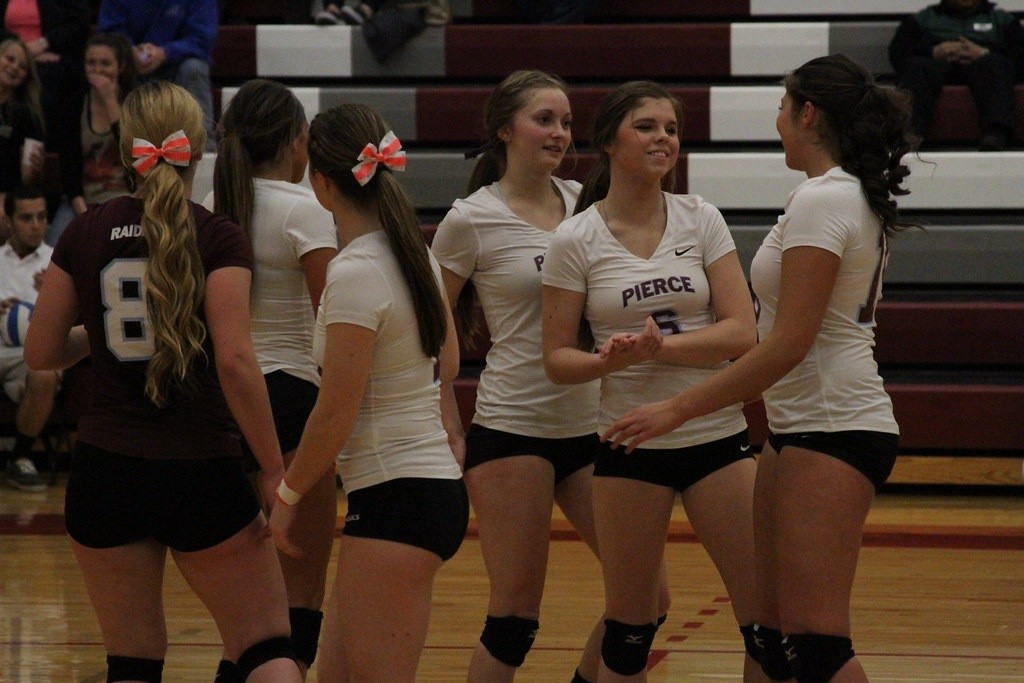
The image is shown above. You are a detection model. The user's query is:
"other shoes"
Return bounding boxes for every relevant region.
[340,6,367,26]
[313,10,336,23]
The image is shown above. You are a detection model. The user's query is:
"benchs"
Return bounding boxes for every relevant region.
[190,0,1024,460]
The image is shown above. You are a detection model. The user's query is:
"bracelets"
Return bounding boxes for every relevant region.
[274,479,301,506]
[982,47,990,56]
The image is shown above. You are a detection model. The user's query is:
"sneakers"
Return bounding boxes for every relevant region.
[7,458,48,490]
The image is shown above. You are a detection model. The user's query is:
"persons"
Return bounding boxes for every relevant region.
[0,0,1024,683]
[600,55,916,683]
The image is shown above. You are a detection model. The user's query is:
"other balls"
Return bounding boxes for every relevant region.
[0,300,35,347]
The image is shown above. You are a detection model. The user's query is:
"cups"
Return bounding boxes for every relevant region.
[22,138,43,165]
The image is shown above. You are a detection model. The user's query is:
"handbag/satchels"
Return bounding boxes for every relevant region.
[365,0,448,62]
